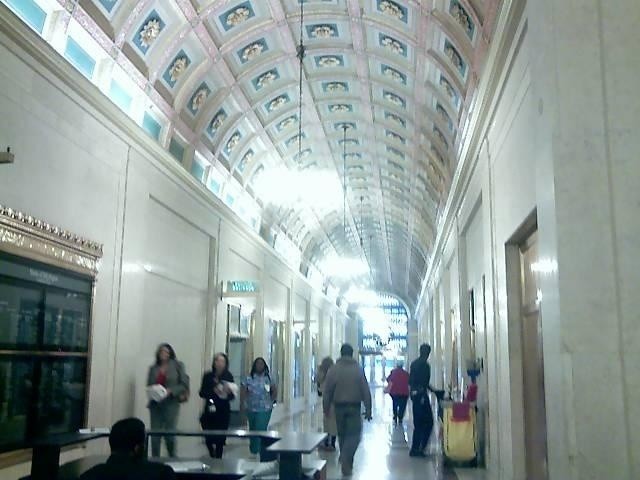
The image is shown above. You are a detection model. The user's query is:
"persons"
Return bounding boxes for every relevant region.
[386,360,410,424]
[408,343,437,458]
[322,343,372,476]
[316,357,338,449]
[198,352,234,459]
[145,343,189,459]
[82,418,176,479]
[241,357,278,458]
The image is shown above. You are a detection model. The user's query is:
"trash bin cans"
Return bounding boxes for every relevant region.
[438,398,478,468]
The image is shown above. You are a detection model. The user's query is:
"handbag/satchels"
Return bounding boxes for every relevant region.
[175,361,189,403]
